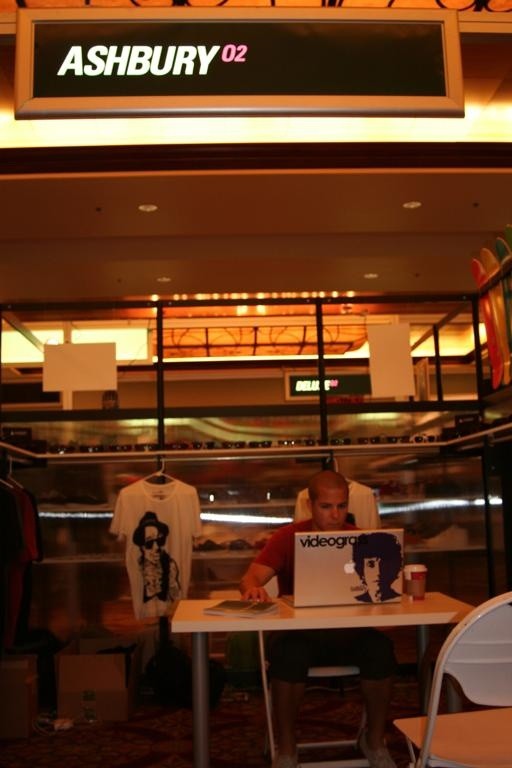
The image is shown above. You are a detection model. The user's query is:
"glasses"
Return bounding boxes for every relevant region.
[356,436,382,444]
[329,438,353,445]
[136,443,160,452]
[140,534,166,549]
[79,444,105,453]
[414,435,436,443]
[384,435,410,443]
[165,443,189,450]
[50,444,75,454]
[191,441,215,449]
[277,440,300,446]
[304,439,327,446]
[110,445,132,452]
[221,441,246,449]
[248,441,273,448]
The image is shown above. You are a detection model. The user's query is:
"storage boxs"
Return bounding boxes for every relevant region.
[1,634,133,744]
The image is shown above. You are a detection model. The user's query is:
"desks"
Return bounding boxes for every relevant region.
[170,588,475,767]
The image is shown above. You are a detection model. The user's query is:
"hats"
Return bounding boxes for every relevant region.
[131,510,170,547]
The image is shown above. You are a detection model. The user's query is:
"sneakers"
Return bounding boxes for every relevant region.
[357,727,398,767]
[191,538,254,551]
[269,746,301,767]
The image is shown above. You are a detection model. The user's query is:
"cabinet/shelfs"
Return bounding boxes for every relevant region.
[2,412,512,572]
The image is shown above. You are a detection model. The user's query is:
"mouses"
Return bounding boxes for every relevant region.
[248,598,267,604]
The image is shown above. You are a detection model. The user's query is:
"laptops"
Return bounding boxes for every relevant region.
[282,527,405,610]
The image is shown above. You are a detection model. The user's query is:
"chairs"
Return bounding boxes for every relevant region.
[390,587,512,767]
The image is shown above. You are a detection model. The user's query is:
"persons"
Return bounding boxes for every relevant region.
[240,469,402,768]
[131,510,181,601]
[352,531,402,602]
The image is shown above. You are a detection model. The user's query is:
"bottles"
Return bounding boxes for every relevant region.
[79,672,97,723]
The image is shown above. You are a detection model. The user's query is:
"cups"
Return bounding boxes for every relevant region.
[403,564,428,603]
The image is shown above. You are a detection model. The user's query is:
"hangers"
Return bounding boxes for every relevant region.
[0,452,23,486]
[140,457,176,489]
